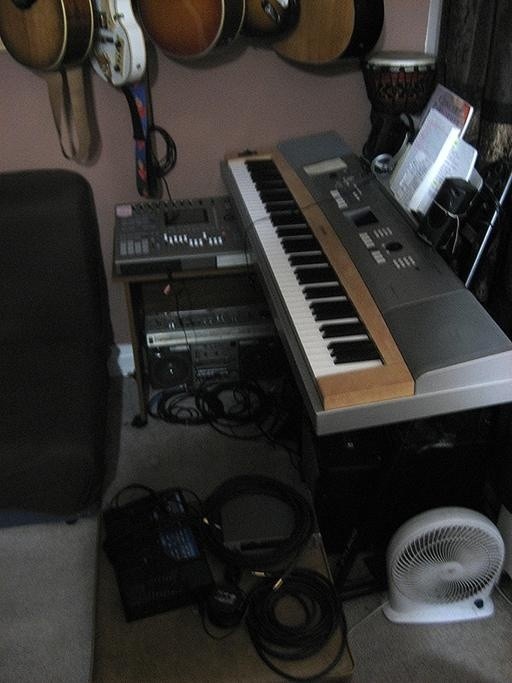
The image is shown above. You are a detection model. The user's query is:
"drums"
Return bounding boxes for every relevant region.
[361,51,436,164]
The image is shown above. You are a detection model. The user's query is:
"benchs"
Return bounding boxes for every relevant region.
[0,169,123,528]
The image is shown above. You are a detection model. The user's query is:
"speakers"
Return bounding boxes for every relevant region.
[417,178,477,248]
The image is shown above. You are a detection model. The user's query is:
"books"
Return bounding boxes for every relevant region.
[448,138,479,181]
[388,107,460,209]
[388,83,473,214]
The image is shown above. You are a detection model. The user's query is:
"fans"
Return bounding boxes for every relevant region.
[379,506,506,625]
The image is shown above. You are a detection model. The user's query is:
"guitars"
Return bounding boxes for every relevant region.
[0,0,383,88]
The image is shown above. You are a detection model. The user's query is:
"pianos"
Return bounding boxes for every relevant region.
[220,129,512,437]
